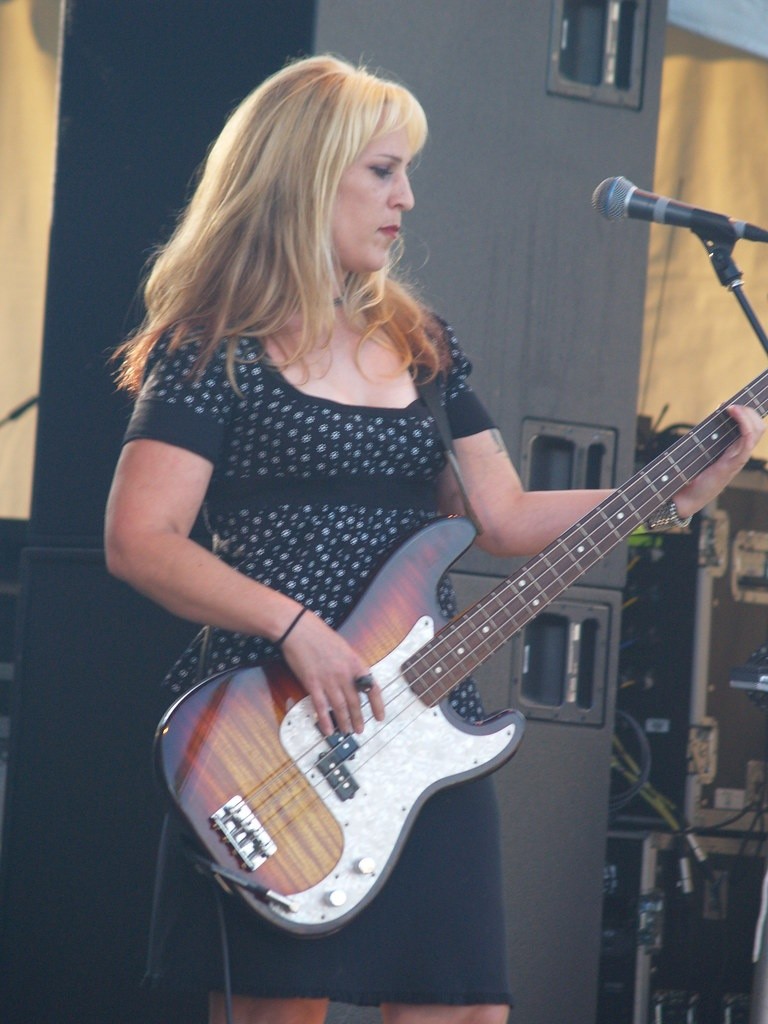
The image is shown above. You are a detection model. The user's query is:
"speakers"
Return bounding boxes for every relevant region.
[28,0,665,591]
[0,546,624,1024]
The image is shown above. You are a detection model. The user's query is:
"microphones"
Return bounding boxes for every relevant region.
[592,176,768,243]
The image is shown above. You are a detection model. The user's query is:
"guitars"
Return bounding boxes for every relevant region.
[146,369,766,939]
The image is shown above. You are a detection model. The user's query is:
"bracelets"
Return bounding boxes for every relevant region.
[642,497,694,529]
[273,608,309,647]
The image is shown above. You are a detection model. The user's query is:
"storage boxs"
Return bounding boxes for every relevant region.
[611,465,768,837]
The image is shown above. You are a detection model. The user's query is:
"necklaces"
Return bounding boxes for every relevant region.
[334,297,344,307]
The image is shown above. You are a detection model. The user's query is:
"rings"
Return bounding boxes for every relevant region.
[357,674,375,690]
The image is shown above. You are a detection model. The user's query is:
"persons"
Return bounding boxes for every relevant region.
[102,57,768,1024]
